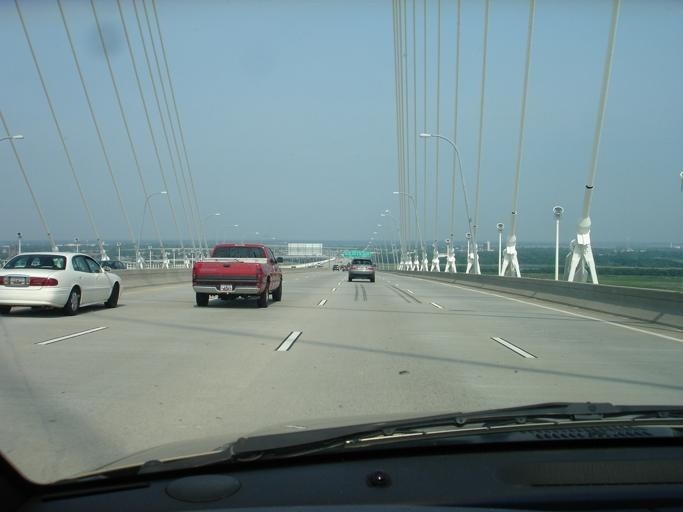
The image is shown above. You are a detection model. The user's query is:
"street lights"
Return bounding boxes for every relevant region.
[136,191,168,269]
[419,134,478,274]
[198,213,220,257]
[365,192,423,270]
[0,135,24,142]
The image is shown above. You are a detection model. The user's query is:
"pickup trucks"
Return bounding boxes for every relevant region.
[192,244,283,308]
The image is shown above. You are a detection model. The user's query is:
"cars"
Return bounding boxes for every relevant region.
[333,259,376,282]
[0,252,122,316]
